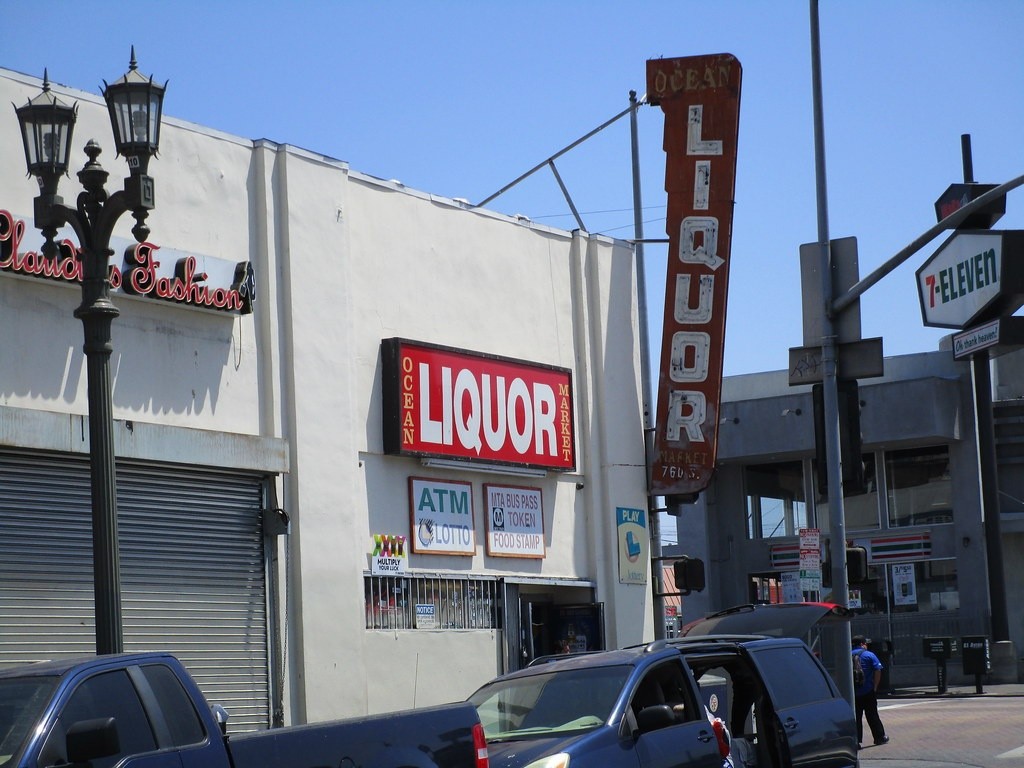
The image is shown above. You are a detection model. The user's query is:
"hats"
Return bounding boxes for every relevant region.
[851,635,872,645]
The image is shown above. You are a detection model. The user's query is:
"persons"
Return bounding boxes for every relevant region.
[850,633,890,748]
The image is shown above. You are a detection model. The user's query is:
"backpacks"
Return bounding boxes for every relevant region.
[853,649,866,689]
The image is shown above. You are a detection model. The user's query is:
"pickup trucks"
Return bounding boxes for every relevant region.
[0,649,488,768]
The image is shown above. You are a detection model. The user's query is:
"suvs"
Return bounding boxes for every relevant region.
[467,601,860,768]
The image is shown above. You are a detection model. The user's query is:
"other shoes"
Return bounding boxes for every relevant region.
[873,735,890,745]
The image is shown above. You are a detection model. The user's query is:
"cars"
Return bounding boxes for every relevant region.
[665,616,681,631]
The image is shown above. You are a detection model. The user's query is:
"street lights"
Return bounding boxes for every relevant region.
[9,40,170,655]
